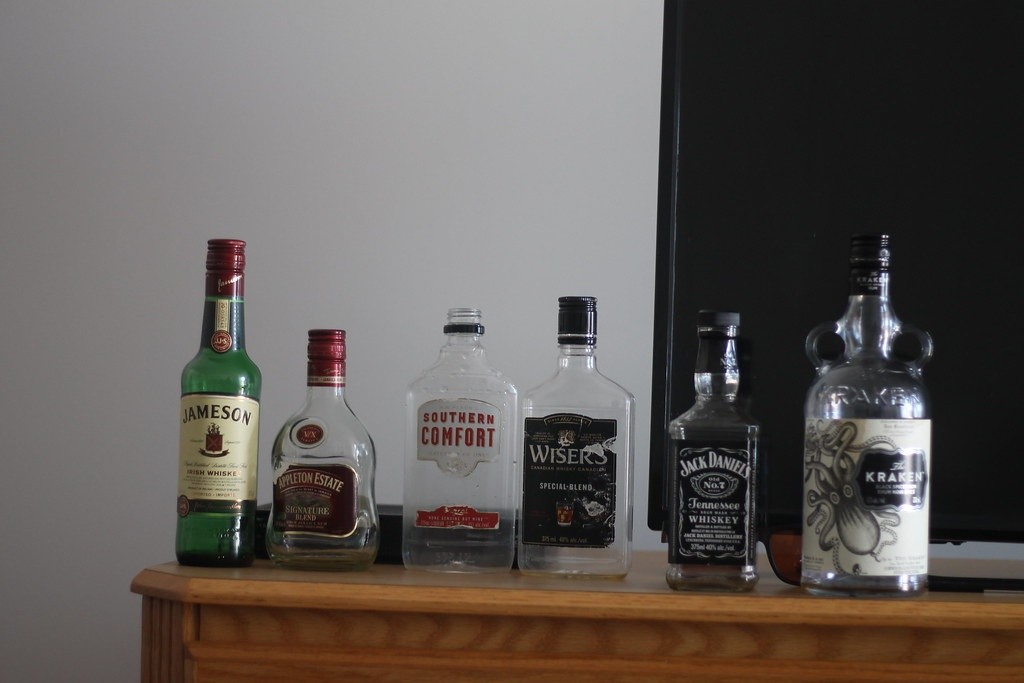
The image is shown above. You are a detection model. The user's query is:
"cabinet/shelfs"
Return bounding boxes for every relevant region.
[130,548,1024,683]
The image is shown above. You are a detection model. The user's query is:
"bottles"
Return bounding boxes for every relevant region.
[176,234,934,599]
